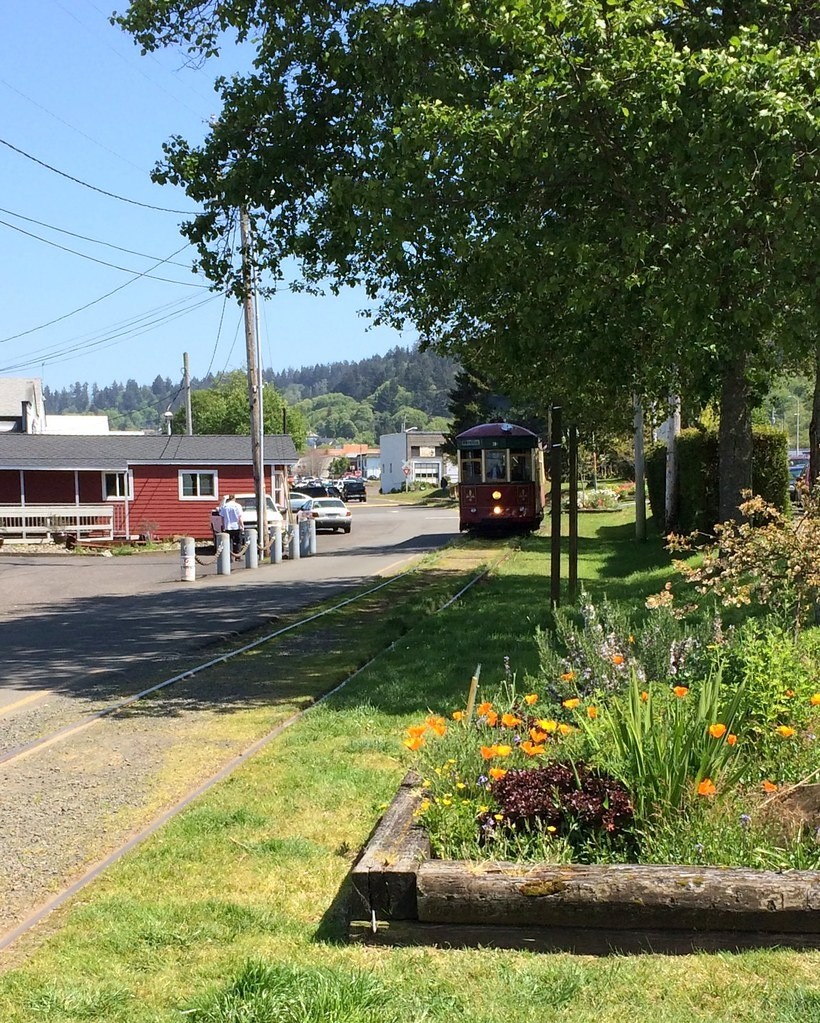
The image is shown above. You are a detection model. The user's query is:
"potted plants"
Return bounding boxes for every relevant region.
[45,514,69,544]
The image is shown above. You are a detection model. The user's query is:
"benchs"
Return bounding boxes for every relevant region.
[0,506,114,545]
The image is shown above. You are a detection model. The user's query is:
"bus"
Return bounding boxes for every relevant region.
[453,423,545,537]
[789,455,810,490]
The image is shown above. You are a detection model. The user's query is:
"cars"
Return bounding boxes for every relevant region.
[296,500,352,534]
[788,465,804,498]
[288,492,321,512]
[288,476,357,499]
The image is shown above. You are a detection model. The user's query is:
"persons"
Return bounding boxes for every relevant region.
[219,494,244,561]
[441,477,448,497]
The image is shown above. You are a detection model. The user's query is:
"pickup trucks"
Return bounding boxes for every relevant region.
[210,493,286,554]
[341,484,366,502]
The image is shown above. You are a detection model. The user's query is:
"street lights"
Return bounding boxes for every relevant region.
[163,412,174,434]
[787,395,799,456]
